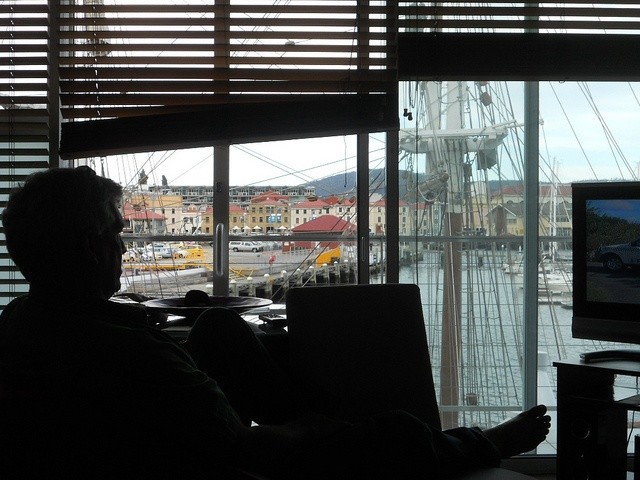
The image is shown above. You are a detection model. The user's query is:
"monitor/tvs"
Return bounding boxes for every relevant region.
[568,180,640,364]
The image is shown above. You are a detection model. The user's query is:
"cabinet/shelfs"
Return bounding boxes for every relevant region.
[552,351,636,480]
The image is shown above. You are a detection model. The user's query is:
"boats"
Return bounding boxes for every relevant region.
[503,249,575,310]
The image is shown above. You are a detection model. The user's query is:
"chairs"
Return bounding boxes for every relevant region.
[286,283,535,480]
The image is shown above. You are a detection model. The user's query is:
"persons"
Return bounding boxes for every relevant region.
[0,165,553,479]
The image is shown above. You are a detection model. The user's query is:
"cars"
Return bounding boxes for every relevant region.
[595,239,640,273]
[122,241,202,262]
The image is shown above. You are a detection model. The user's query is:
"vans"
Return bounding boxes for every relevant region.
[230,241,262,253]
